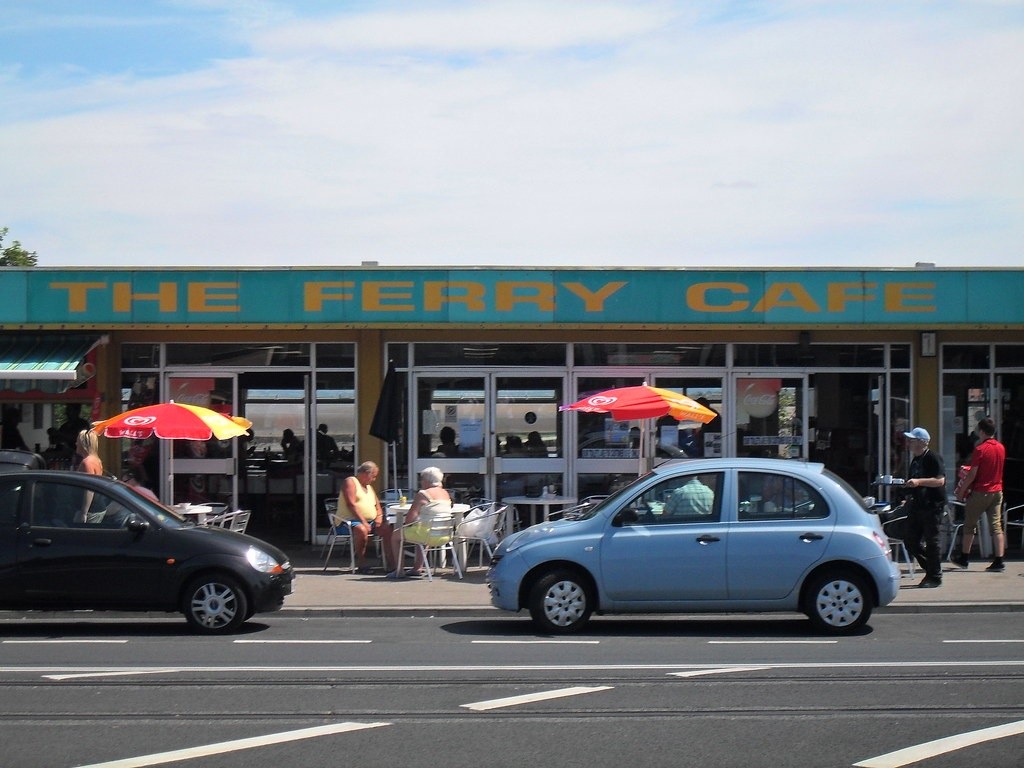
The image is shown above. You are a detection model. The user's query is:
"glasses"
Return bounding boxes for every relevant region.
[904,437,918,443]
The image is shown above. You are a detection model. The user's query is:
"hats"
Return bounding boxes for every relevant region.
[904,427,930,441]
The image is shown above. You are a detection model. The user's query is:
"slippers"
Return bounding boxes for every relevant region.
[386,570,405,578]
[406,568,423,576]
[358,566,372,575]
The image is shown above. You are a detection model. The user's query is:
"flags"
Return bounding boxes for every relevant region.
[370,360,404,447]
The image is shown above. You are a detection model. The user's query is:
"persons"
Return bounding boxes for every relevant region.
[316,423,349,464]
[44,402,90,459]
[386,466,453,582]
[902,425,949,588]
[280,429,304,460]
[694,397,722,457]
[660,472,715,516]
[523,429,549,459]
[432,426,474,484]
[332,458,394,572]
[0,407,31,451]
[237,428,257,464]
[950,416,1006,573]
[497,434,543,497]
[71,428,109,524]
[466,430,503,496]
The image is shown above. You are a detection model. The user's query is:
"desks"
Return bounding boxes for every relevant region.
[175,505,213,524]
[502,495,578,526]
[227,475,335,494]
[388,503,470,575]
[636,501,665,515]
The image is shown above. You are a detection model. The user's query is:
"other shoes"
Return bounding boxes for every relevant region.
[952,558,968,569]
[986,563,1006,572]
[918,575,942,588]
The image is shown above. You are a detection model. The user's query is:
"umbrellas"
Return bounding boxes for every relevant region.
[558,381,717,477]
[91,396,254,506]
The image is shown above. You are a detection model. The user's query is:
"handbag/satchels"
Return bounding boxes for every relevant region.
[75,470,117,513]
[459,503,501,548]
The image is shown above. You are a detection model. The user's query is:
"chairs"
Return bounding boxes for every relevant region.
[197,460,354,534]
[947,501,1024,563]
[546,495,611,521]
[882,509,916,580]
[319,488,523,582]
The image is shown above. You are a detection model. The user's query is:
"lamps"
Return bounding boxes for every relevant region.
[798,332,815,359]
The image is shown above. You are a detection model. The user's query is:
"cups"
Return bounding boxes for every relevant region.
[399,496,406,507]
[178,503,192,510]
[874,474,905,484]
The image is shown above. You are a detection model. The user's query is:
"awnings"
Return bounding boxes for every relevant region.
[0,332,111,381]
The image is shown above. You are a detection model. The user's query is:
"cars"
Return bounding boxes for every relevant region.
[485,457,901,635]
[501,430,687,526]
[0,471,296,635]
